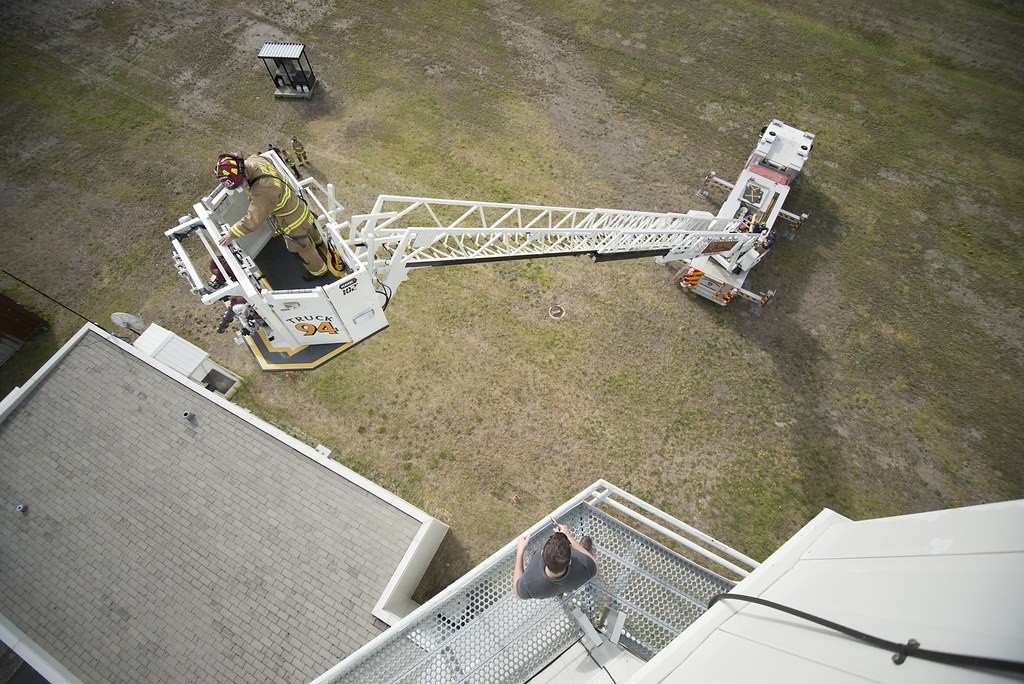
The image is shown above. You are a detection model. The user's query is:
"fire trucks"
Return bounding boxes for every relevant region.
[164,117,817,349]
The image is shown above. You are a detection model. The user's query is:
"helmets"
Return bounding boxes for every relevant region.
[215,152,244,190]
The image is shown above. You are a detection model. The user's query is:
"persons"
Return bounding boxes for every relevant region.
[513,523,600,600]
[282,150,301,179]
[291,136,309,167]
[268,144,281,154]
[214,152,327,281]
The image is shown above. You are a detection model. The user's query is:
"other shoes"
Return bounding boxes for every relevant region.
[302,270,326,280]
[299,163,303,167]
[297,175,300,178]
[579,536,592,551]
[306,161,309,164]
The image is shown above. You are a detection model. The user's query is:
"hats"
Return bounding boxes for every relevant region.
[291,136,298,142]
